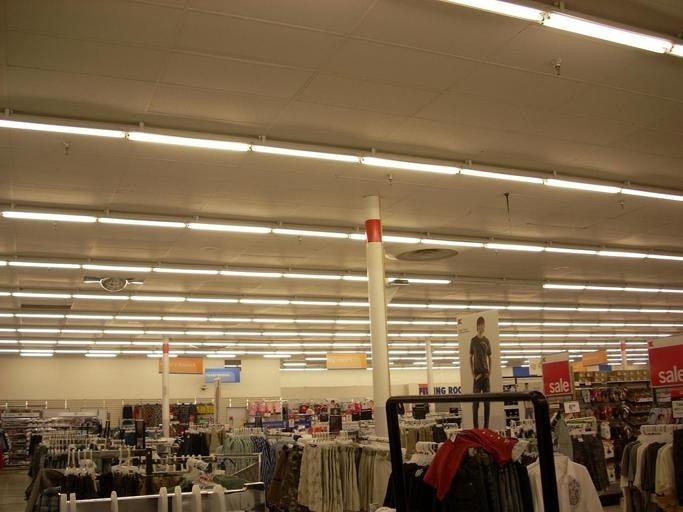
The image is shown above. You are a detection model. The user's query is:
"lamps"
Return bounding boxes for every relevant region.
[450,1,683,59]
[0,110,682,370]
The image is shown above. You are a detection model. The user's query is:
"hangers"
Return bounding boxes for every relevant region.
[36,417,683,512]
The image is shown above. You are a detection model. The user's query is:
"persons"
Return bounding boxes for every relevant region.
[469,316,492,429]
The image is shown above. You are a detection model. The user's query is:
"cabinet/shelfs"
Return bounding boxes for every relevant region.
[502,380,651,426]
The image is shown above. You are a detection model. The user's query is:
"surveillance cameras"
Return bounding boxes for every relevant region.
[200,387,209,391]
